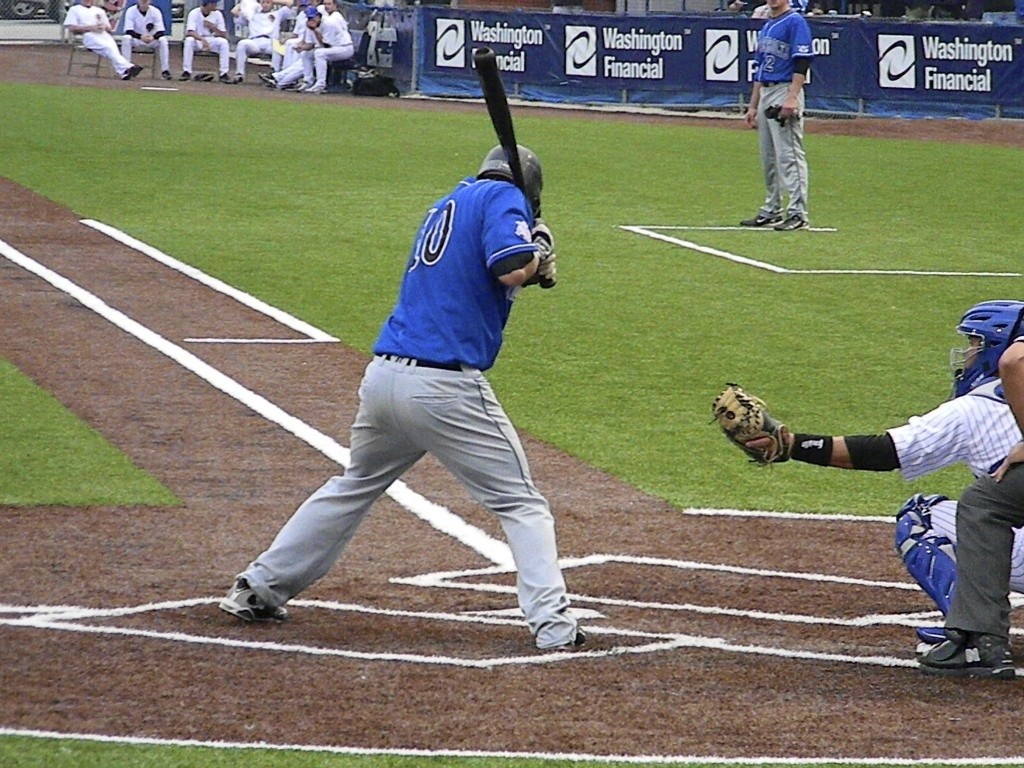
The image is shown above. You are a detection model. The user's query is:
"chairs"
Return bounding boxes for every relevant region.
[62,1,378,93]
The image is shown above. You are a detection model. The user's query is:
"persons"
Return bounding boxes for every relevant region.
[711,300,1023,680]
[215,141,587,651]
[62,0,354,94]
[740,0,811,231]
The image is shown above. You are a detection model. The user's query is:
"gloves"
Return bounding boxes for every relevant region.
[536,254,558,290]
[531,217,555,259]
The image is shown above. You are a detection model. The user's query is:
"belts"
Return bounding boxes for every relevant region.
[374,352,463,372]
[760,80,792,87]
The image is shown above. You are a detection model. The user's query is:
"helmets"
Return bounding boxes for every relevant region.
[949,299,1024,399]
[475,143,543,205]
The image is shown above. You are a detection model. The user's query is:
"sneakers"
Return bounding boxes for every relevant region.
[916,629,1017,681]
[218,73,231,84]
[232,75,244,84]
[257,72,277,87]
[120,65,145,80]
[537,628,589,654]
[161,70,171,80]
[739,213,785,229]
[304,85,324,93]
[294,83,310,92]
[218,577,290,624]
[773,216,809,231]
[178,71,191,83]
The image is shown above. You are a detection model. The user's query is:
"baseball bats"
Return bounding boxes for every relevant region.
[473,45,557,291]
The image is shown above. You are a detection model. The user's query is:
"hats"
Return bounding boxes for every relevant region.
[304,5,318,17]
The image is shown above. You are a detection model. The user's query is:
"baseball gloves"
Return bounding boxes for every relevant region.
[710,385,792,468]
[194,72,214,82]
